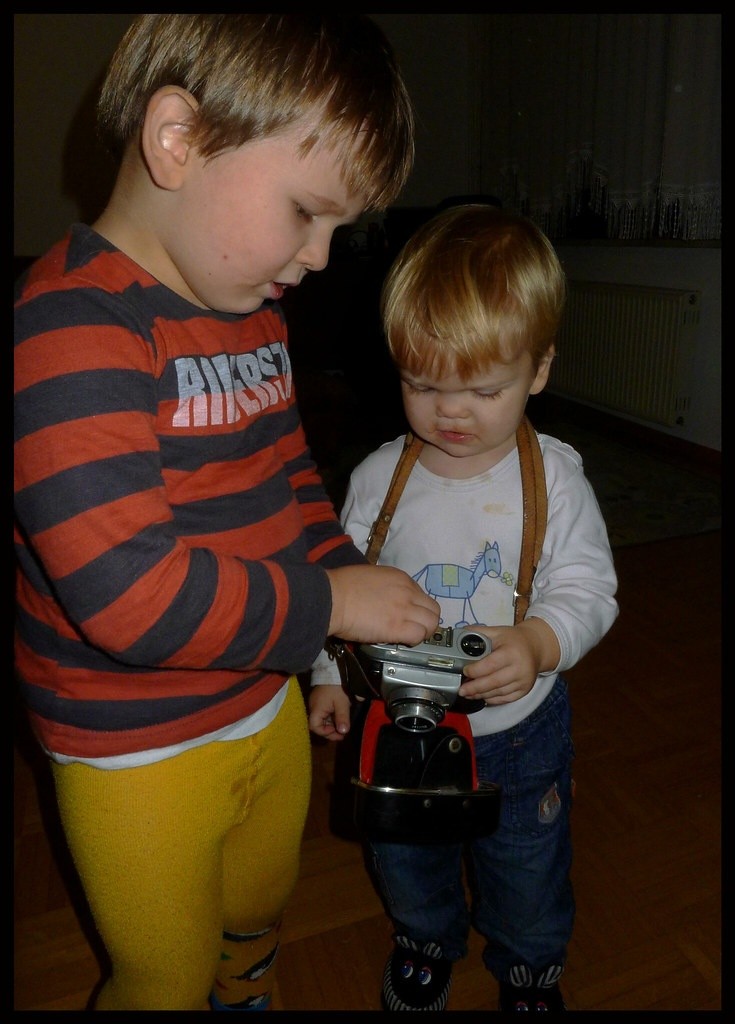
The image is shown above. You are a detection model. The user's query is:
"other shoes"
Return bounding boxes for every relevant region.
[500,947,566,1011]
[383,935,453,1011]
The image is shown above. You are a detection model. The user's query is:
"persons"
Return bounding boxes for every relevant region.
[309,205,618,1011]
[11,14,440,1010]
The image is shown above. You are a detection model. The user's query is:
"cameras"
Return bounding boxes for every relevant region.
[356,626,492,732]
